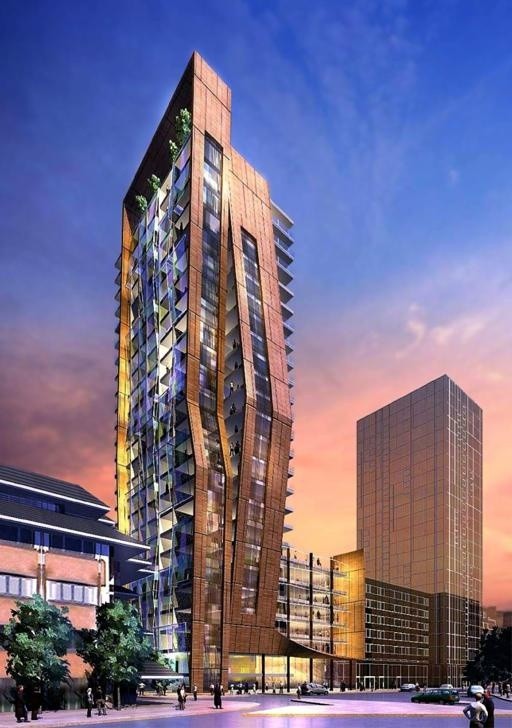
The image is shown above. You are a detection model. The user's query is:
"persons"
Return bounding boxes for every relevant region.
[272,682,276,693]
[86,688,94,717]
[278,681,284,695]
[296,682,302,699]
[178,686,188,710]
[163,682,168,695]
[95,686,108,715]
[139,681,146,695]
[479,688,494,728]
[193,682,198,701]
[209,681,258,697]
[30,686,42,720]
[156,681,164,696]
[14,684,30,722]
[213,682,224,709]
[177,685,182,695]
[463,692,488,728]
[266,681,269,691]
